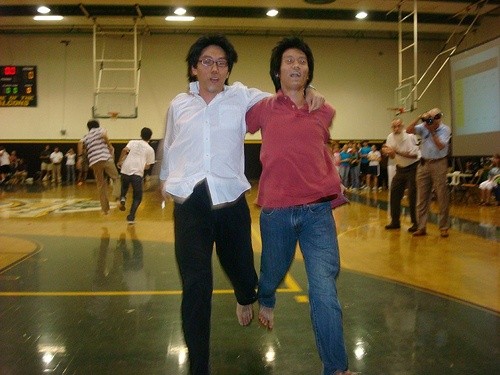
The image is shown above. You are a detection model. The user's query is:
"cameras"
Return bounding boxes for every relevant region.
[422,118,433,125]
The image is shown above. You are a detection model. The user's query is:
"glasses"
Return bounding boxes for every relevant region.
[199,58,227,67]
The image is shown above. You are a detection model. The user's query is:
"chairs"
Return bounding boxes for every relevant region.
[459,169,485,205]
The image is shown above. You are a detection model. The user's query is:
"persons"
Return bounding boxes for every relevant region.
[381,117,420,232]
[160,33,325,375]
[82,120,120,216]
[117,126,156,224]
[328,140,500,206]
[0,145,89,186]
[245,36,357,375]
[406,108,453,238]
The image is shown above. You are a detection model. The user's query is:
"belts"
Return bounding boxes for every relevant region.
[311,193,337,205]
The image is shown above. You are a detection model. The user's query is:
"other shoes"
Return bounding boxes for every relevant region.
[413,231,426,235]
[472,201,490,207]
[127,215,134,224]
[119,197,126,211]
[408,227,417,232]
[385,225,400,229]
[441,231,448,237]
